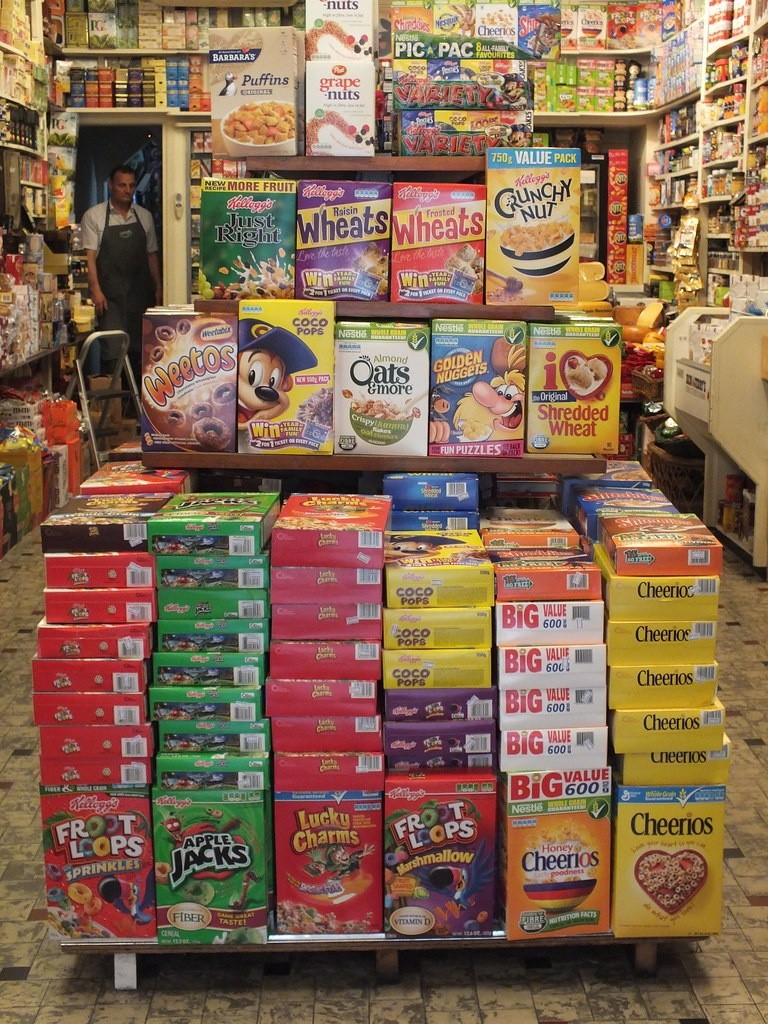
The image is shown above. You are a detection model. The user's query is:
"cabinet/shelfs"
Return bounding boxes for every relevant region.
[651,0,768,318]
[51,0,310,114]
[0,0,95,380]
[515,0,707,130]
[141,154,607,474]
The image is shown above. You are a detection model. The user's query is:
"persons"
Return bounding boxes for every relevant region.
[79,163,162,418]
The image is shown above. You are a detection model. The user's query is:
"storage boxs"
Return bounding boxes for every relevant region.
[33,0,731,946]
[0,390,54,561]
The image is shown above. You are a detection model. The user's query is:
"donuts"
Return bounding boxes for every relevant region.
[194,418,232,450]
[166,409,185,425]
[192,403,212,420]
[214,383,236,403]
